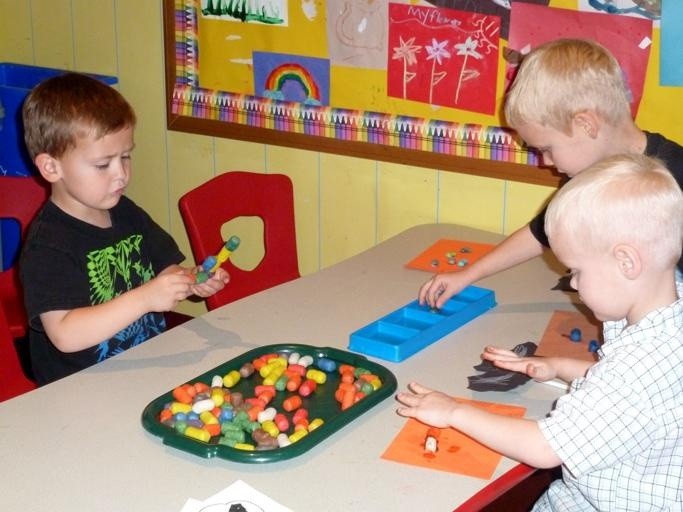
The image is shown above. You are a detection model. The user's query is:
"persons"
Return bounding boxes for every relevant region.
[419,39,683,310]
[396,151,683,512]
[17,73,231,388]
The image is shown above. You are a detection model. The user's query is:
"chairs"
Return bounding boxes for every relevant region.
[175,168,304,312]
[0,174,53,411]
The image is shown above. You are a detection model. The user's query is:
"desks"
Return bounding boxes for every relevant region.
[0,221,607,512]
